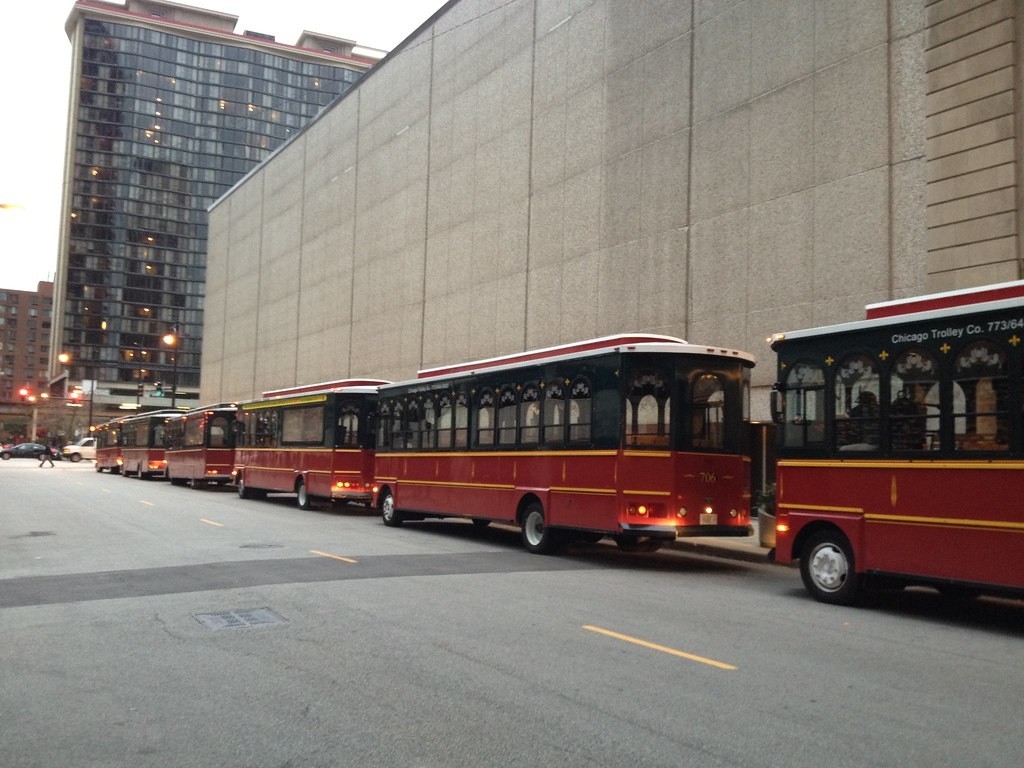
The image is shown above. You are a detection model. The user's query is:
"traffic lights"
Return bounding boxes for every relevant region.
[156,382,163,396]
[137,384,144,397]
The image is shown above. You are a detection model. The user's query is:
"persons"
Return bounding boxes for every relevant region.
[848,392,879,417]
[39,443,55,468]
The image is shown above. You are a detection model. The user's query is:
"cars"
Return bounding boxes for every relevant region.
[0,438,96,463]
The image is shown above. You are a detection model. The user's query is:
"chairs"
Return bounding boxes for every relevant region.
[846,391,927,451]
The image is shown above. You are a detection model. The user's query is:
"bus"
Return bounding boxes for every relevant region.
[374,332,757,554]
[770,277,1024,605]
[232,378,394,510]
[122,410,187,480]
[93,415,136,474]
[164,402,235,489]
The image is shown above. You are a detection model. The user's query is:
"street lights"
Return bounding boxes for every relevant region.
[20,381,94,438]
[56,350,141,438]
[164,319,180,410]
